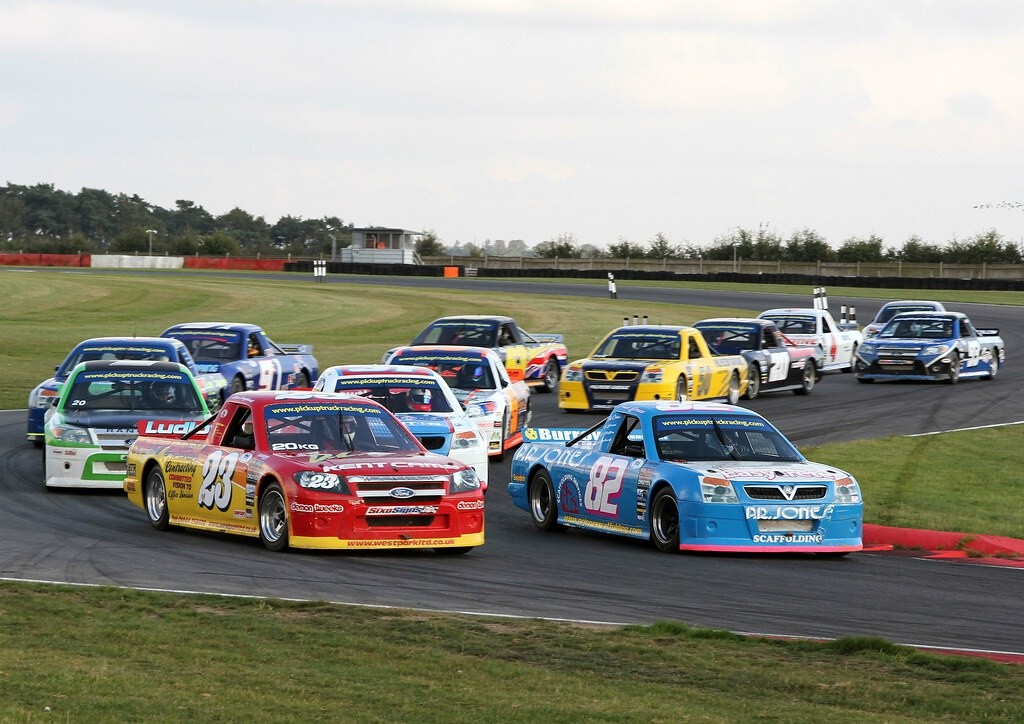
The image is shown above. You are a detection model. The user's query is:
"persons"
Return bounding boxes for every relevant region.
[802,322,816,334]
[149,381,177,408]
[940,323,962,337]
[227,336,258,359]
[456,364,486,388]
[704,430,739,458]
[670,341,690,359]
[401,388,433,411]
[321,415,357,450]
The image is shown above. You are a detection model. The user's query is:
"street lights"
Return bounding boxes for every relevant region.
[732,243,742,272]
[697,254,703,273]
[145,229,158,256]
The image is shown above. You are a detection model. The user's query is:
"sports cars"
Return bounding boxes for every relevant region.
[43,358,214,490]
[852,311,1005,386]
[381,345,532,463]
[409,315,571,394]
[122,386,486,556]
[507,397,866,556]
[25,336,229,443]
[756,306,864,377]
[556,323,751,413]
[293,360,490,492]
[690,319,825,401]
[159,320,320,399]
[859,302,953,339]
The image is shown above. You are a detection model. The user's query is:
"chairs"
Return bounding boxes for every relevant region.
[316,417,337,449]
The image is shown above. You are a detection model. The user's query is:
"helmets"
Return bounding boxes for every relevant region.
[460,363,486,385]
[406,387,433,412]
[671,342,691,356]
[704,430,740,456]
[322,415,359,445]
[805,322,816,332]
[150,380,177,406]
[942,322,953,335]
[149,353,170,362]
[485,327,503,342]
[247,337,253,351]
